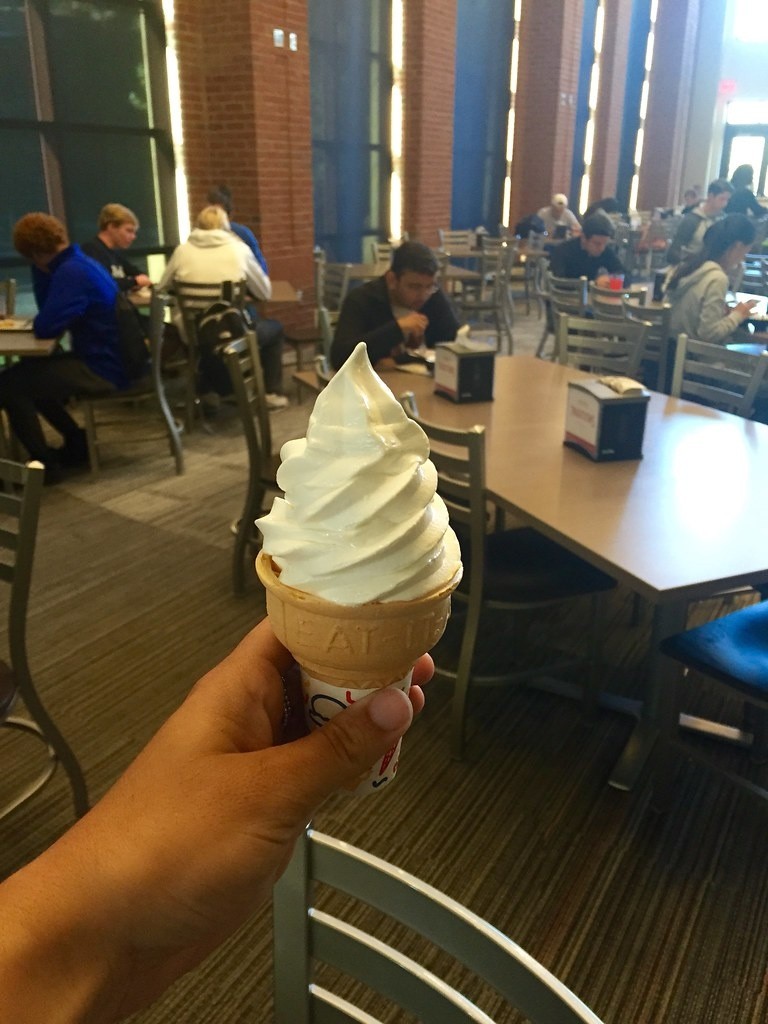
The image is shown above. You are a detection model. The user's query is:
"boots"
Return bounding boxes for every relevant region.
[55,429,88,464]
[30,449,64,484]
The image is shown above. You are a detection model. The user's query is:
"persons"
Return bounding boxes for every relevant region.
[329,241,462,373]
[92,188,289,409]
[0,614,435,1024]
[537,165,768,427]
[0,213,131,490]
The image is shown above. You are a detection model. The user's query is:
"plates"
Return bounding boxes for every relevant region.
[0,319,34,331]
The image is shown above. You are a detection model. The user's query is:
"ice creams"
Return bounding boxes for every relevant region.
[253,338,463,794]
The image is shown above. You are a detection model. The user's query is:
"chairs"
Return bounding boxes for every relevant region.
[224,332,285,601]
[403,411,618,767]
[72,284,185,477]
[1,458,91,823]
[170,207,768,432]
[272,824,613,1024]
[647,598,768,816]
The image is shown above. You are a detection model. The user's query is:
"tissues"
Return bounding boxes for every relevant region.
[563,375,652,461]
[434,324,498,403]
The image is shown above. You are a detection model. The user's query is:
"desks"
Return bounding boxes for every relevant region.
[289,347,768,794]
[0,313,66,465]
[130,273,296,306]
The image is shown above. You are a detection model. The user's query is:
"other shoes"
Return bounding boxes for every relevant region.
[265,394,290,408]
[200,390,221,413]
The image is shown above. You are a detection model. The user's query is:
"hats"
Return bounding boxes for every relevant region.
[551,194,568,207]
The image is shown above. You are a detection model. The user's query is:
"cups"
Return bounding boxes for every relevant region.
[608,272,625,290]
